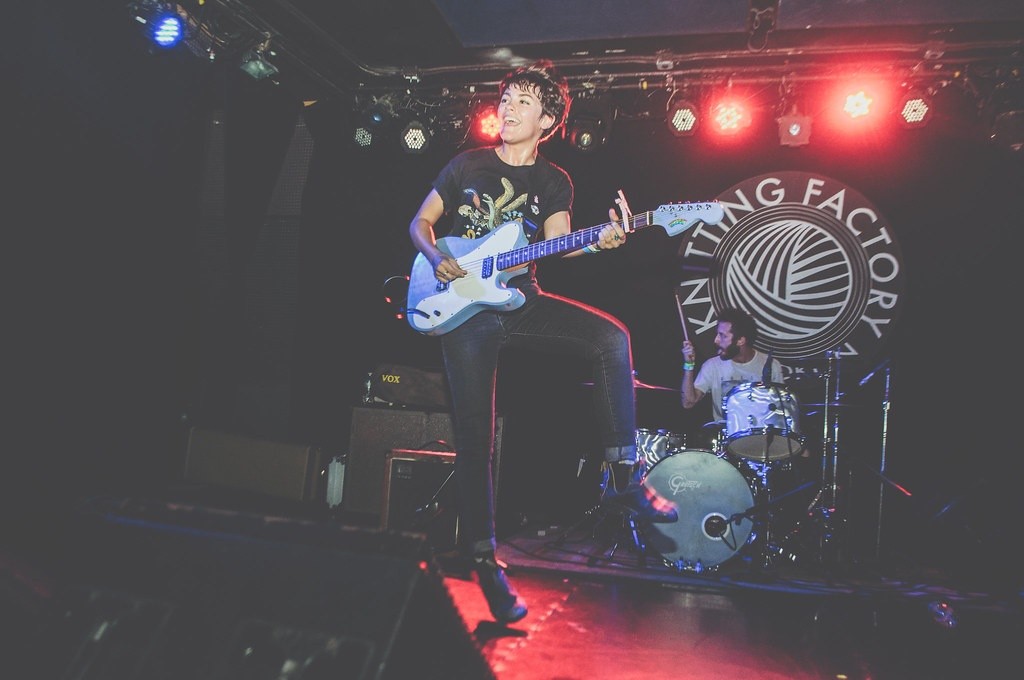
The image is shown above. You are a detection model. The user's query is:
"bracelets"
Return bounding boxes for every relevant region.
[683,362,696,371]
[582,245,601,254]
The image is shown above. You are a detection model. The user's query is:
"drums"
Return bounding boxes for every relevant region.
[633,424,690,476]
[635,446,763,573]
[723,378,808,464]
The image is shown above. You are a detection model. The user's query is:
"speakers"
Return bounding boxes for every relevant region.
[0,406,509,680]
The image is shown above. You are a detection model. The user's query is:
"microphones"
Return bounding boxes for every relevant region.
[858,360,890,386]
[763,354,773,382]
[708,521,723,533]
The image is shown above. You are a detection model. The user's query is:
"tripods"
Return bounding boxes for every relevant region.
[742,356,860,572]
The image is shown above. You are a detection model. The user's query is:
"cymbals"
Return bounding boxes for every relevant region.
[578,377,687,394]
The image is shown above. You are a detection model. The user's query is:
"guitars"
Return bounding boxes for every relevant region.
[404,196,726,339]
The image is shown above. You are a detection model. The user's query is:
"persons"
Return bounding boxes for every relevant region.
[408,63,680,622]
[680,309,785,451]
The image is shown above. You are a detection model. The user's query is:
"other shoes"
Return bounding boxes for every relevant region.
[601,460,679,524]
[474,560,527,624]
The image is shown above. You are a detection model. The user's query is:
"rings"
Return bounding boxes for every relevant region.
[441,271,448,277]
[611,234,619,241]
